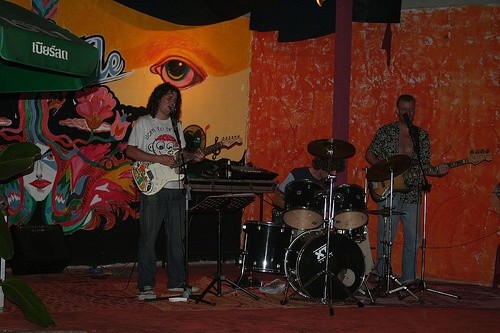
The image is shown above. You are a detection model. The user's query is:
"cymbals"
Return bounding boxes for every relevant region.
[368,209,406,218]
[365,153,414,183]
[306,138,357,161]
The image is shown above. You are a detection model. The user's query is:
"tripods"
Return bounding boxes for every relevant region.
[279,156,365,314]
[142,111,216,306]
[186,192,261,305]
[356,130,462,308]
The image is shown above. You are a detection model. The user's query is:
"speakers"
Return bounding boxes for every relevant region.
[8,223,75,277]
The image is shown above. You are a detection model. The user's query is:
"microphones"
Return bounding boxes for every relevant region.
[402,112,416,136]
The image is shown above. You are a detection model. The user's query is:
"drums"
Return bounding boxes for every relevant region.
[284,228,368,304]
[329,183,368,232]
[238,219,295,276]
[281,178,326,231]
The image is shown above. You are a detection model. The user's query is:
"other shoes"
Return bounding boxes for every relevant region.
[140,289,154,295]
[365,273,377,290]
[399,290,431,300]
[168,285,199,293]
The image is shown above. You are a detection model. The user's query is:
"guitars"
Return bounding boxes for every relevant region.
[132,135,244,197]
[368,148,493,202]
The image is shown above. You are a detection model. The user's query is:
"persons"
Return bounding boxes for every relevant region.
[363,95,450,289]
[273,156,343,206]
[125,83,204,297]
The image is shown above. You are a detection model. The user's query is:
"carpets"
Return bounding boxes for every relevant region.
[5,276,500,312]
